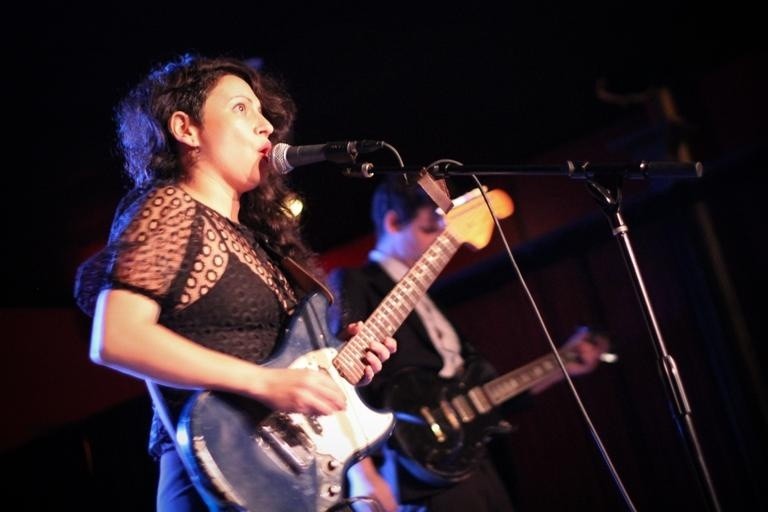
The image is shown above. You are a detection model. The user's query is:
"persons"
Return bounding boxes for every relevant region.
[75,53,397,512]
[326,174,515,511]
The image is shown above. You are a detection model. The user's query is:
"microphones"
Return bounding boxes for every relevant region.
[265,141,355,175]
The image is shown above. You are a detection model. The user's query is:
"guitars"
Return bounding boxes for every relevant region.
[176,187,514,512]
[387,326,613,483]
